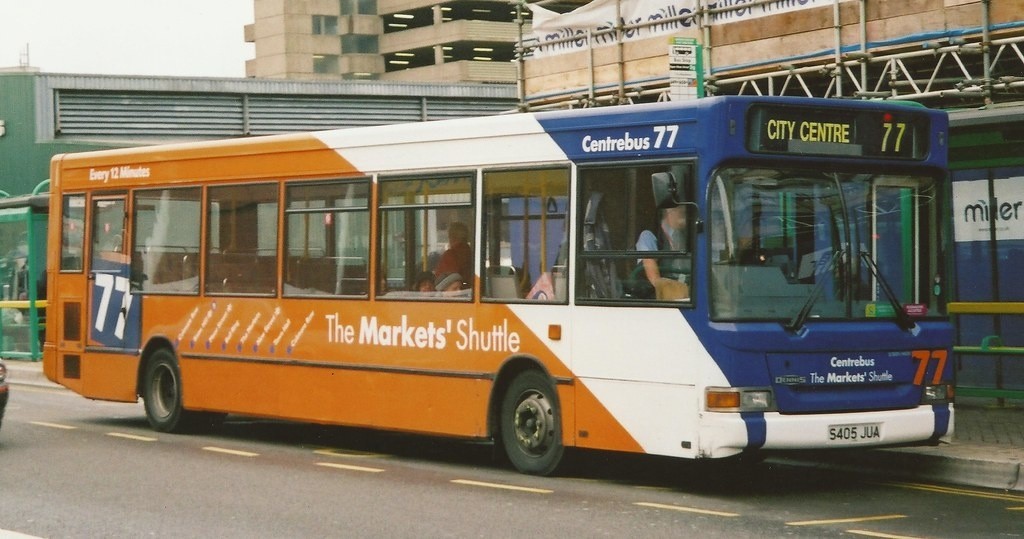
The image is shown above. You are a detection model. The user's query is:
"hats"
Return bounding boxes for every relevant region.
[435,272,462,291]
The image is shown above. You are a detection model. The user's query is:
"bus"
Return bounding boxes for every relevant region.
[40,95,958,479]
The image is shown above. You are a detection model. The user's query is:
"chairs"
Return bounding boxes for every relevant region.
[376,287,414,299]
[60,246,369,299]
[486,263,524,301]
[551,265,569,302]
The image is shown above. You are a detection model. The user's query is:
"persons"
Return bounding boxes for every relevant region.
[634,196,689,297]
[433,222,472,286]
[434,272,464,291]
[412,271,436,292]
